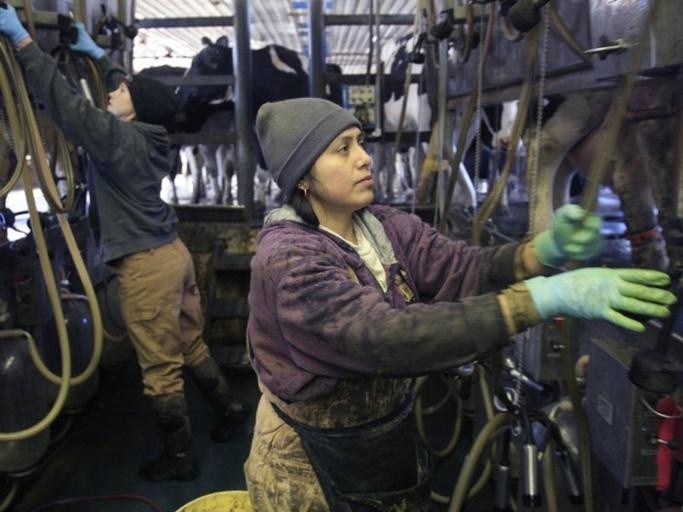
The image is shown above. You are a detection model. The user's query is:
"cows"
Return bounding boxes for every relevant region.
[135,35,341,207]
[373,2,683,273]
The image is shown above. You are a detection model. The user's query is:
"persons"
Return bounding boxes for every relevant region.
[0,1,254,478]
[242,96,678,511]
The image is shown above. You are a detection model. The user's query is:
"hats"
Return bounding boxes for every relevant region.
[254,96,360,198]
[126,71,178,122]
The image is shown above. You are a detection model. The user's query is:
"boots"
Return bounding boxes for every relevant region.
[138,393,199,482]
[190,360,255,443]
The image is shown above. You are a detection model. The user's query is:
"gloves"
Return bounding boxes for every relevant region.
[0,5,28,47]
[67,21,105,62]
[526,264,677,335]
[532,204,607,269]
